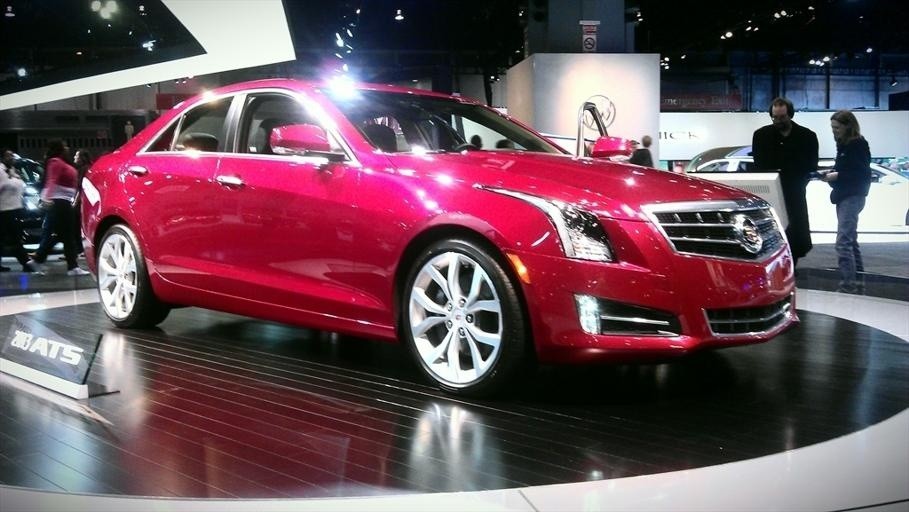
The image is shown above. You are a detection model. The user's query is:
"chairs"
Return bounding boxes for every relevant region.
[364,124,397,152]
[183,132,218,151]
[255,118,293,153]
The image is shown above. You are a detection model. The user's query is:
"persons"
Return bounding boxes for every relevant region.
[496,139,514,148]
[751,97,819,278]
[0,148,36,273]
[26,138,90,276]
[816,109,872,273]
[73,148,92,261]
[629,135,654,167]
[471,134,482,150]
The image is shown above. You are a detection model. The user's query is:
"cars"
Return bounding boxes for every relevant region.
[688,155,756,174]
[797,159,908,232]
[72,76,801,397]
[13,156,61,245]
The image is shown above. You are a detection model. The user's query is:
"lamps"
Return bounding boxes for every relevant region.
[5,0,15,18]
[394,0,404,20]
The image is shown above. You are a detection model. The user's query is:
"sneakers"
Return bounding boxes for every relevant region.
[66,268,92,277]
[21,263,46,276]
[0,265,11,273]
